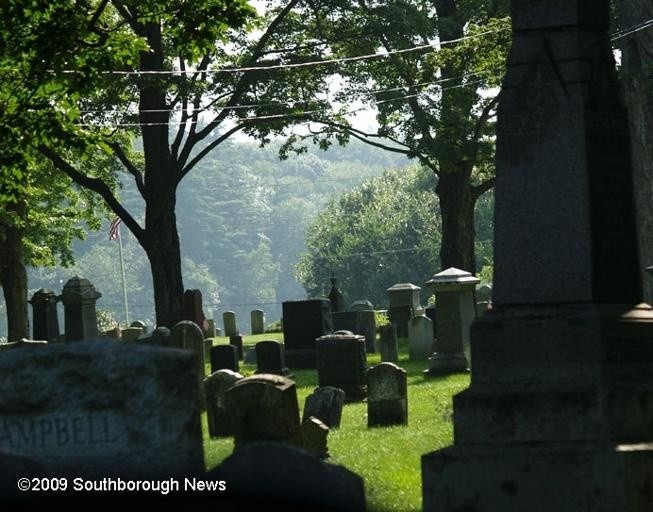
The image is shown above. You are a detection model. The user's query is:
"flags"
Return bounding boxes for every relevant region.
[109,217,122,240]
